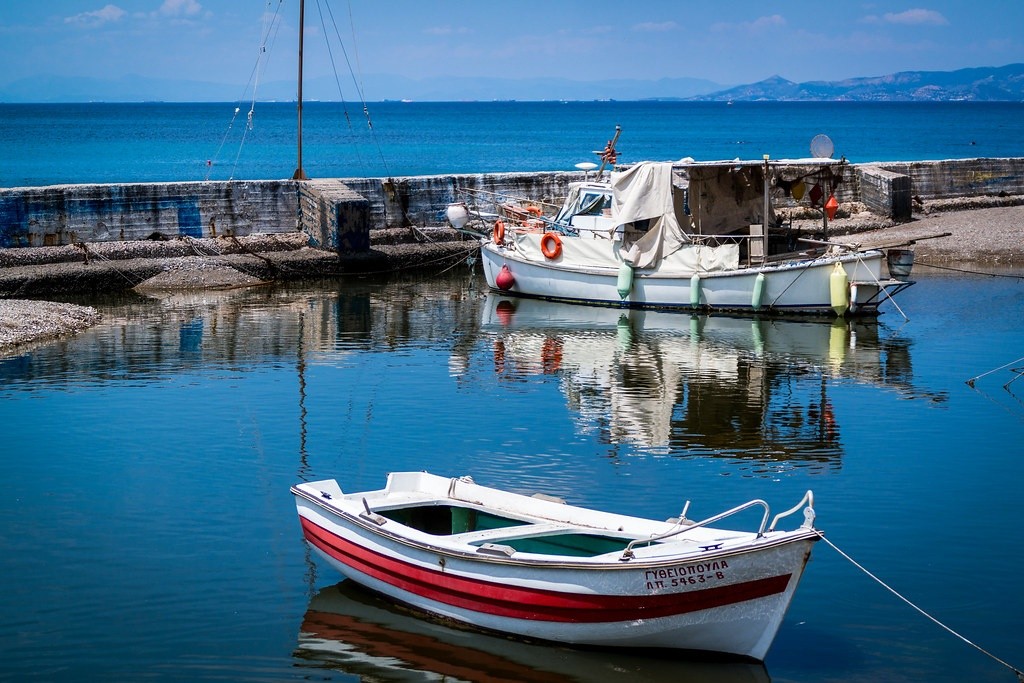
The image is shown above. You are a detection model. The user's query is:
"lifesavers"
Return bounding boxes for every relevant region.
[523,206,542,229]
[540,232,563,258]
[494,220,505,245]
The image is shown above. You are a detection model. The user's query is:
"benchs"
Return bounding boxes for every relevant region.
[444,523,576,544]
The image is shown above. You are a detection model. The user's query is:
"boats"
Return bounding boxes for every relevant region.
[480,123,917,314]
[289,464,824,665]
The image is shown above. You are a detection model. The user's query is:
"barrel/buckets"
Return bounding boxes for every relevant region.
[887,249,914,281]
